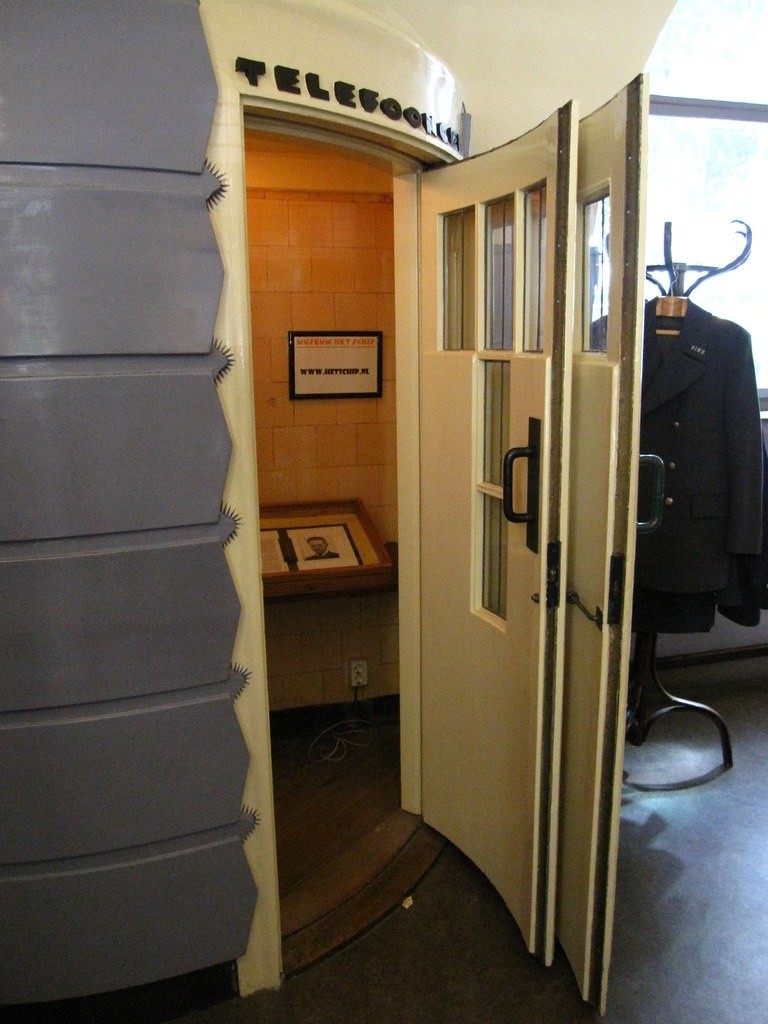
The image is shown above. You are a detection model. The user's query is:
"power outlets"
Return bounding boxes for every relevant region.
[350,658,368,687]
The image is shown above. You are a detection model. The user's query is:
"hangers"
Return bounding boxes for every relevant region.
[654,268,688,336]
